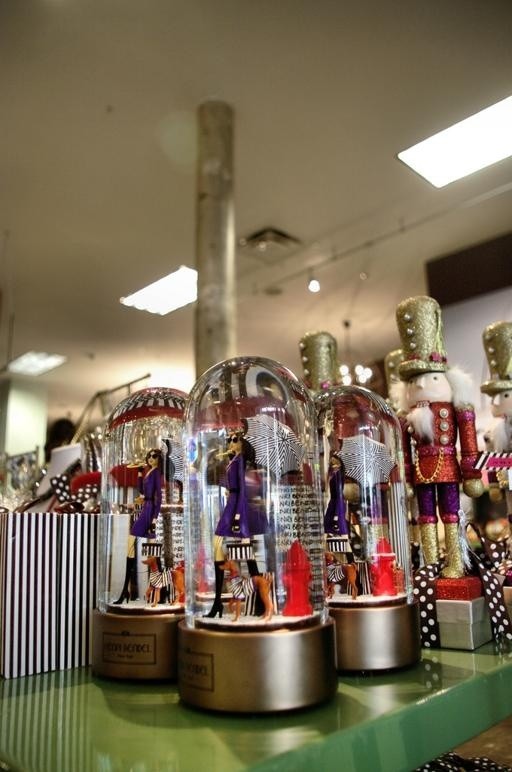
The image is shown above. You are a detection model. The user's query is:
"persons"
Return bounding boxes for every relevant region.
[43,420,79,463]
[88,292,512,715]
